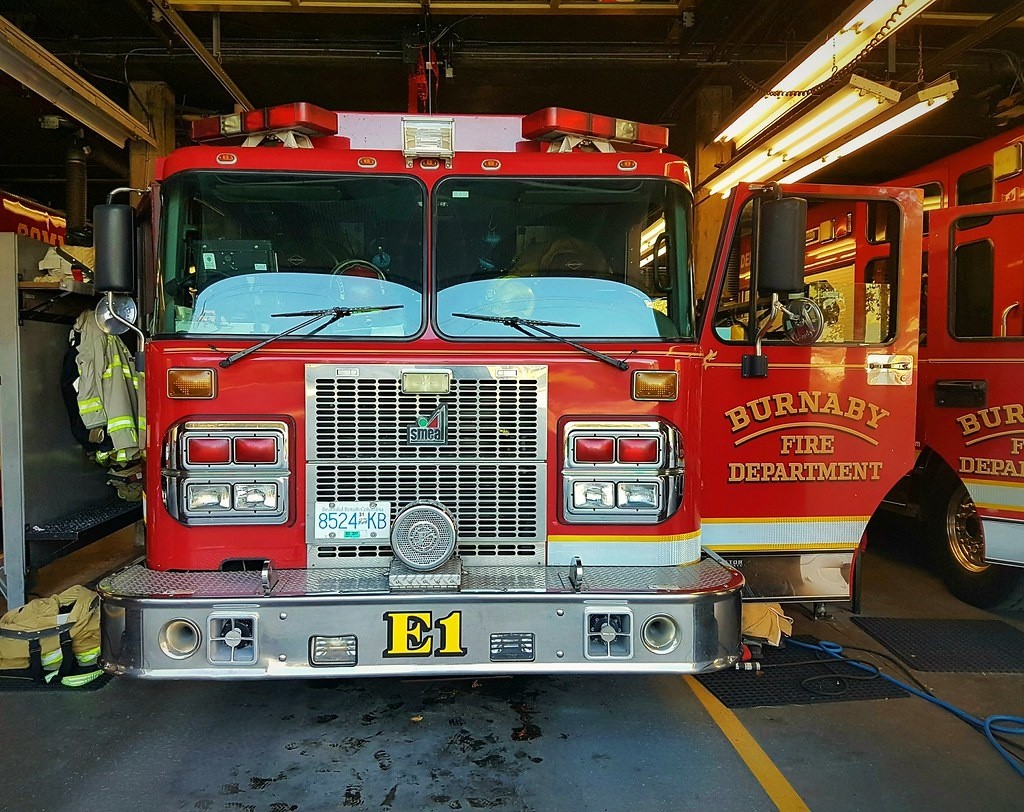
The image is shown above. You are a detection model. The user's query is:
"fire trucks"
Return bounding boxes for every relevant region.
[717,126,1024,612]
[92,99,925,679]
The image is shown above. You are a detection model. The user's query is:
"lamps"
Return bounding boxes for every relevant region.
[700,1,960,209]
